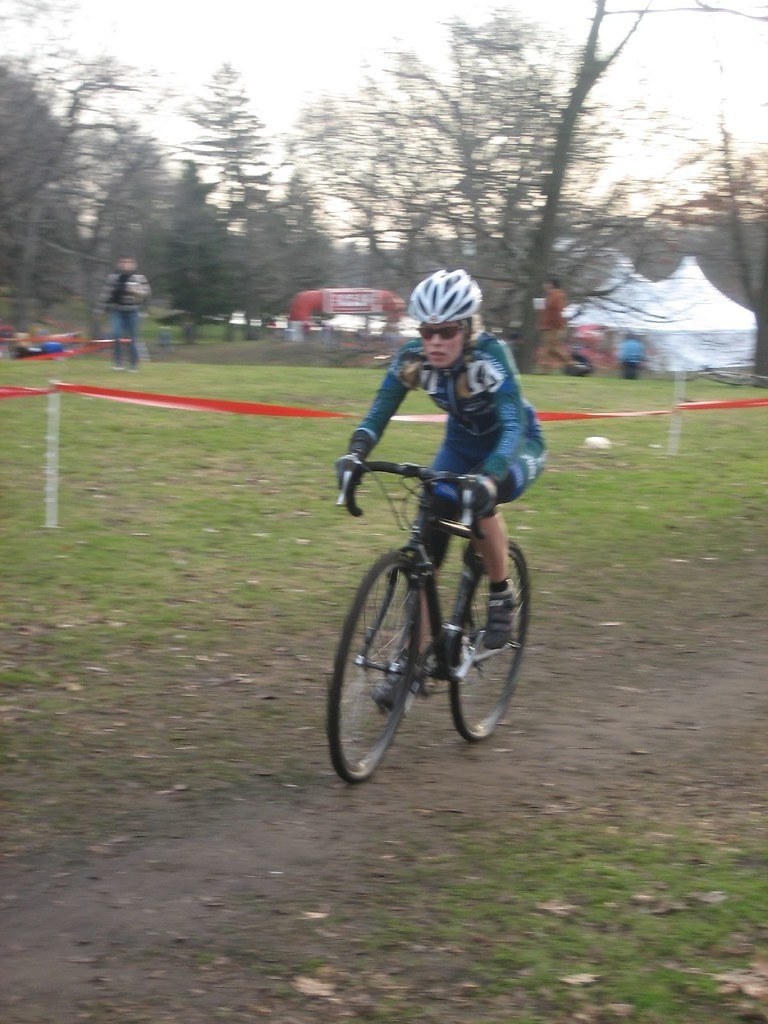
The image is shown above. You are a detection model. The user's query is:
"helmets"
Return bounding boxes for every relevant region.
[409,269,483,324]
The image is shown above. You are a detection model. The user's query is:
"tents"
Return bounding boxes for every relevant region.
[533,248,757,371]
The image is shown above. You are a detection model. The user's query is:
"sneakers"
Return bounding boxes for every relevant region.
[483,580,515,649]
[372,648,422,706]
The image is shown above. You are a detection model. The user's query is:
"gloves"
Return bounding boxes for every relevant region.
[335,453,370,489]
[462,470,499,520]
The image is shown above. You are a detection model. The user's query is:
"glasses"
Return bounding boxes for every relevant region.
[416,325,464,340]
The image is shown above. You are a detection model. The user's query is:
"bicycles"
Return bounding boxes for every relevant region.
[325,460,531,785]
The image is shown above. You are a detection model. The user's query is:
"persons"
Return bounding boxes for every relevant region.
[335,268,548,709]
[532,277,646,379]
[93,253,152,372]
